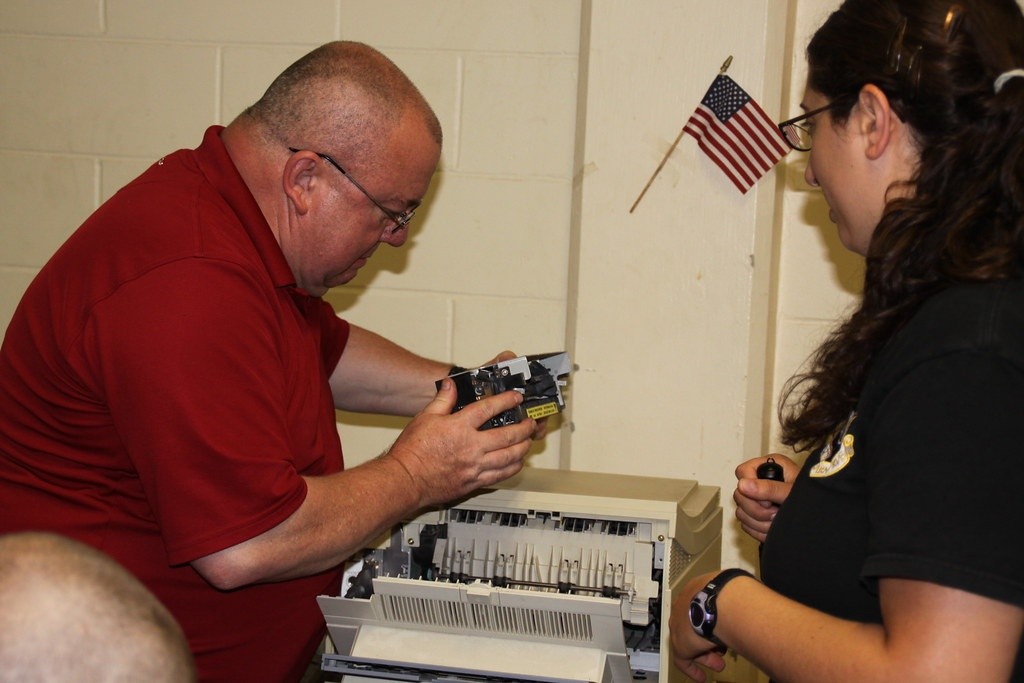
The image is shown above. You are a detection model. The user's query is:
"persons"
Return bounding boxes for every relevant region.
[1,534,189,683]
[668,0,1024,683]
[0,39,568,683]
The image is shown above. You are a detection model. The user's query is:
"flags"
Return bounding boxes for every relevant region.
[685,75,800,196]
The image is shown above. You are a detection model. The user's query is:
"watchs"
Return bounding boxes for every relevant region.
[688,568,762,645]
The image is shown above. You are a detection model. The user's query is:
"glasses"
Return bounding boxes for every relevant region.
[779,94,906,153]
[289,143,416,234]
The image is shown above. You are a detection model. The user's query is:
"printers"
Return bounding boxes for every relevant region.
[317,465,724,683]
